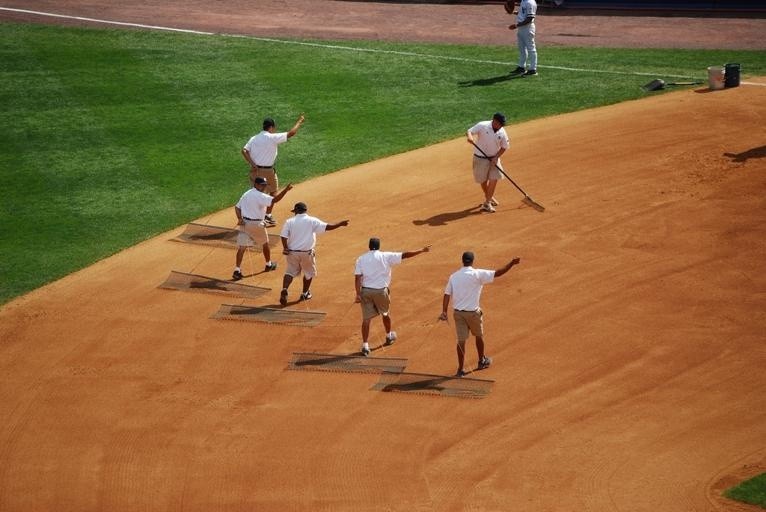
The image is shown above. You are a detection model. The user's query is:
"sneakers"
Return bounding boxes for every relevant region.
[481,200,496,213]
[361,346,370,356]
[264,263,277,272]
[456,368,467,377]
[490,196,499,206]
[264,215,277,224]
[509,67,527,75]
[477,355,491,370]
[521,69,538,77]
[299,291,313,300]
[386,331,396,345]
[232,271,244,279]
[280,288,288,306]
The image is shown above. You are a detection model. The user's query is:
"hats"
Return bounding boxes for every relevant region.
[369,238,380,250]
[254,176,268,185]
[290,202,308,212]
[463,251,474,263]
[492,112,506,126]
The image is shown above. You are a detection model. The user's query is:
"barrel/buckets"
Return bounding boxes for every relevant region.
[707,65,725,89]
[725,63,740,88]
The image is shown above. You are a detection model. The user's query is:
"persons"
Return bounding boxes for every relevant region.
[355,238,433,355]
[507,0,538,75]
[243,115,305,227]
[466,113,510,212]
[279,203,349,305]
[233,176,292,279]
[439,251,520,378]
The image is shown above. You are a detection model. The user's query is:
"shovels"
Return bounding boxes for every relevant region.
[470,140,545,214]
[643,77,704,91]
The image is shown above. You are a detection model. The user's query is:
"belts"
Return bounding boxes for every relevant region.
[257,165,273,169]
[474,153,493,159]
[243,217,262,221]
